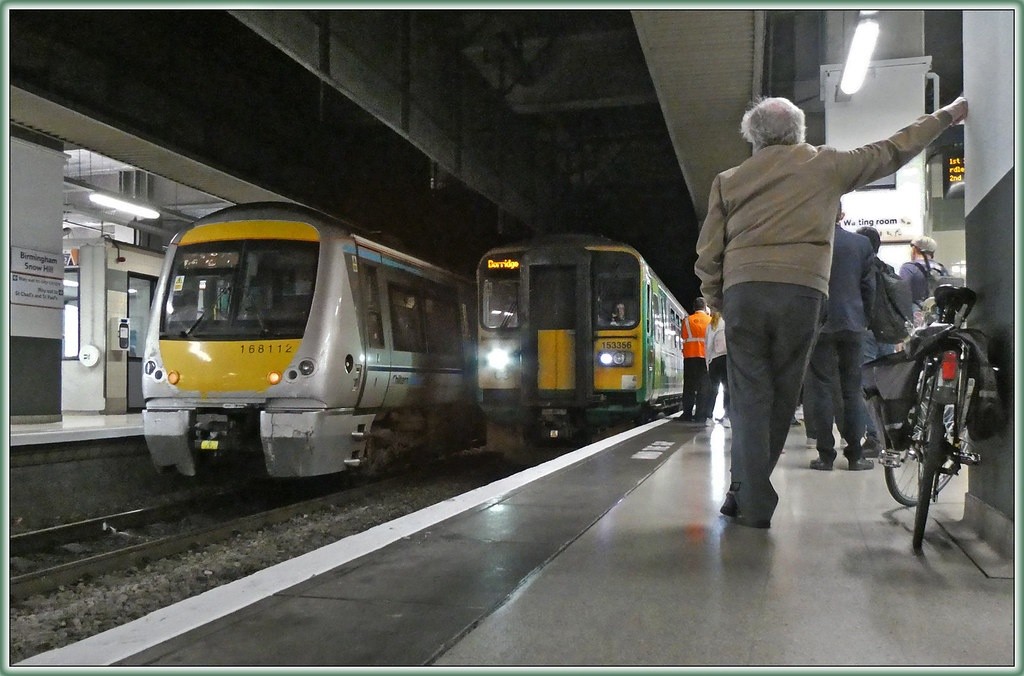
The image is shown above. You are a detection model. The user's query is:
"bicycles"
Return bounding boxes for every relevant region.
[878,283,982,550]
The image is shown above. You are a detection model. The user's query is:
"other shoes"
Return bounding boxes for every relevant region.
[806,438,817,448]
[695,416,706,422]
[848,456,874,470]
[706,417,715,426]
[861,441,880,458]
[673,413,691,420]
[719,504,772,528]
[809,457,833,470]
[839,438,848,450]
[722,418,731,427]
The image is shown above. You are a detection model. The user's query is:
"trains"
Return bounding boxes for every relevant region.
[140,199,486,502]
[475,236,691,467]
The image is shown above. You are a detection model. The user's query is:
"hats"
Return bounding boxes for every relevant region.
[911,235,937,253]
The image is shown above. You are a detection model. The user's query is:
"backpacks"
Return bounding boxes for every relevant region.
[868,263,912,344]
[909,259,945,300]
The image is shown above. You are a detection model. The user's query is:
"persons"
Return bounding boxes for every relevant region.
[899,237,955,325]
[697,95,955,527]
[673,297,717,422]
[803,196,875,470]
[851,225,901,457]
[598,302,635,328]
[704,307,735,428]
